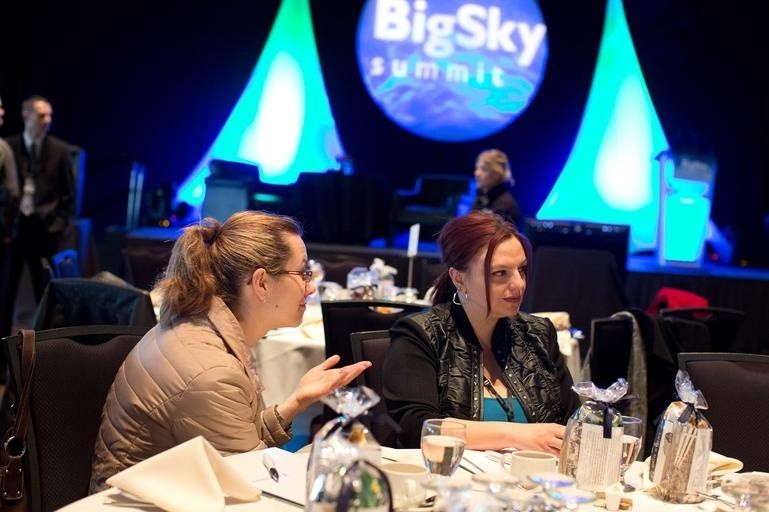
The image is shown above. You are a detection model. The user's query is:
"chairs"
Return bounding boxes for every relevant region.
[675,350,769,472]
[2,321,154,512]
[350,330,396,438]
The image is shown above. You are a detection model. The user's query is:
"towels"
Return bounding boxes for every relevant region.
[104,434,263,512]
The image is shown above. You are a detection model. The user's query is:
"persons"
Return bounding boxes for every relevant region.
[1,96,25,395]
[88,208,373,496]
[5,100,77,384]
[371,208,586,451]
[468,147,526,232]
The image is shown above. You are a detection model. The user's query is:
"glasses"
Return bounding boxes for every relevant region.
[245,264,313,285]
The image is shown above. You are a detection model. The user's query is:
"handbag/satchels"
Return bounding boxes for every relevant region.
[575,310,645,463]
[0,324,36,512]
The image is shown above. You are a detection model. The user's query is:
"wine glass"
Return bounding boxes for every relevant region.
[416,473,598,512]
[618,415,644,492]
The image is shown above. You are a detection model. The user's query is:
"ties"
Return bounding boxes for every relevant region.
[28,142,39,176]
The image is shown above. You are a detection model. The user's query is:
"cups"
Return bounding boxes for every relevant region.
[500,449,560,481]
[379,463,431,512]
[603,489,624,511]
[420,418,468,480]
